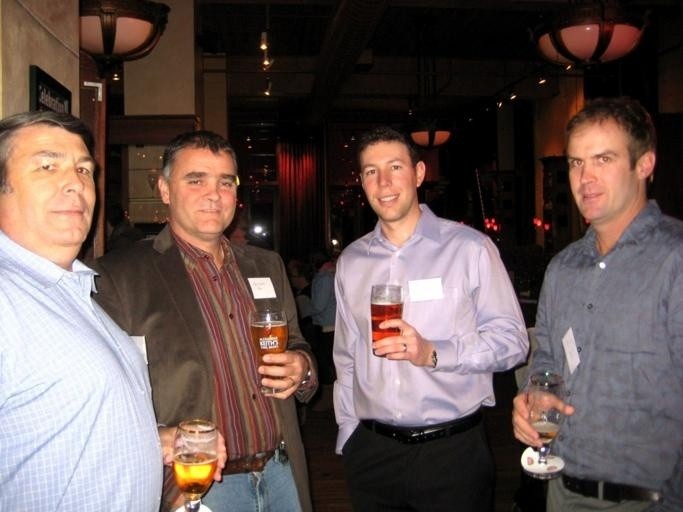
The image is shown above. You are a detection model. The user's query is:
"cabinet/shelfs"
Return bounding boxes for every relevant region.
[119,144,169,228]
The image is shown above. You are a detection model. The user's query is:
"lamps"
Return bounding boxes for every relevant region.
[78,0,171,79]
[410,118,452,147]
[538,0,642,70]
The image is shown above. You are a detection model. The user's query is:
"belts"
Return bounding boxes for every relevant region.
[367,410,481,444]
[216,450,276,479]
[562,474,657,503]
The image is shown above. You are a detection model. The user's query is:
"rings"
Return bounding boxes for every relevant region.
[287,377,295,386]
[402,343,407,353]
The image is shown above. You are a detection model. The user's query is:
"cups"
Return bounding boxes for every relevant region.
[250,311,290,394]
[370,284,404,359]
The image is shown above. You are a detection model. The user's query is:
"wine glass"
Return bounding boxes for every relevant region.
[172,417,219,512]
[519,371,567,480]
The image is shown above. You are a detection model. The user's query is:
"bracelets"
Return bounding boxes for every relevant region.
[299,361,311,390]
[431,350,437,368]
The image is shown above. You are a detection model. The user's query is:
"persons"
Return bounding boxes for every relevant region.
[304,251,337,386]
[0,107,228,512]
[80,132,321,511]
[509,98,683,510]
[330,127,532,511]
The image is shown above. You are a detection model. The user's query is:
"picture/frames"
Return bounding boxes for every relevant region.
[28,65,71,114]
